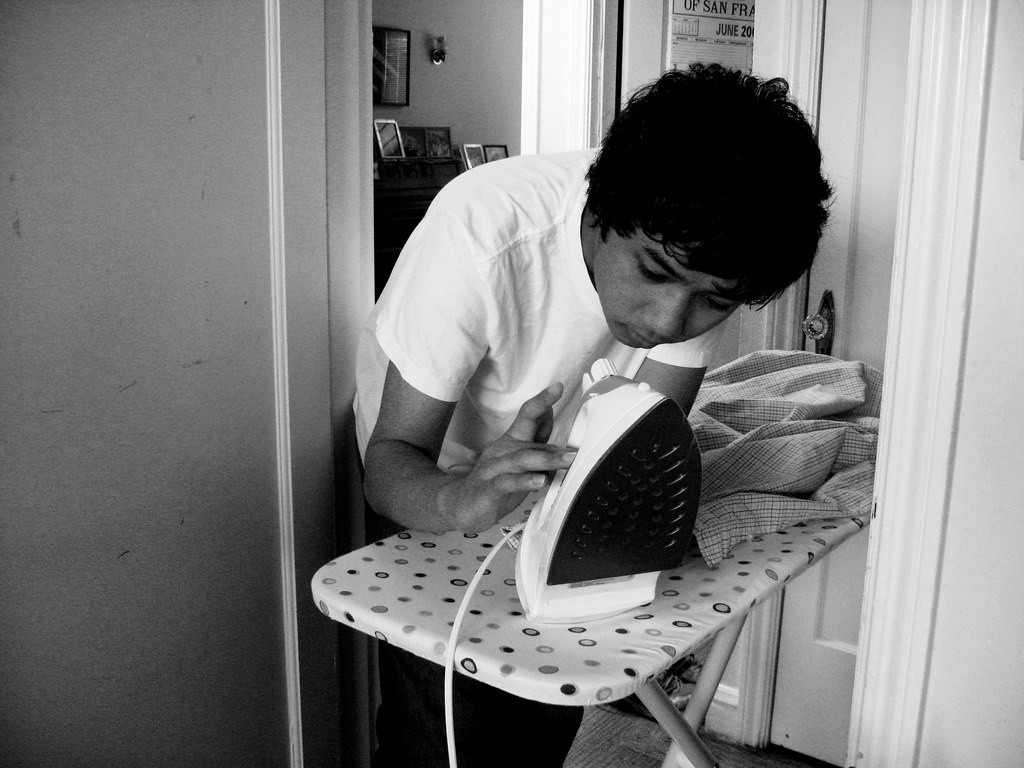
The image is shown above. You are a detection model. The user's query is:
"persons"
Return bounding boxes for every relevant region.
[352,62,834,768]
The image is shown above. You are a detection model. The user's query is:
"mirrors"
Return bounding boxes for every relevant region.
[372,25,411,106]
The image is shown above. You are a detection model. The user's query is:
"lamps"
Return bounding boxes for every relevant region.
[430,36,446,65]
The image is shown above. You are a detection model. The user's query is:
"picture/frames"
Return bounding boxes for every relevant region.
[373,119,509,172]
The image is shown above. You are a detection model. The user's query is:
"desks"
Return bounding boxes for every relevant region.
[310,509,872,767]
